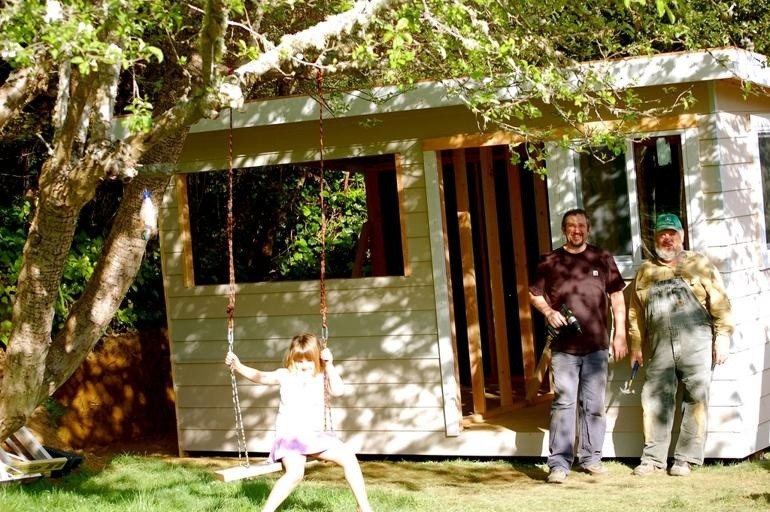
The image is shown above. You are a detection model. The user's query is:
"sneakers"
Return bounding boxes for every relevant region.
[633,461,664,476]
[670,459,691,476]
[547,468,566,483]
[583,460,607,477]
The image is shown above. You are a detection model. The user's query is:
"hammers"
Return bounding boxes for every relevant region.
[620,360,639,395]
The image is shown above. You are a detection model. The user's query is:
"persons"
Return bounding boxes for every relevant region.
[627,212,735,478]
[224,334,373,512]
[528,208,629,485]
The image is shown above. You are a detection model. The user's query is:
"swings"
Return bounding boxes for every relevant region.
[214,73,329,482]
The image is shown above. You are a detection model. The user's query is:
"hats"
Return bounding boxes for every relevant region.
[655,213,682,233]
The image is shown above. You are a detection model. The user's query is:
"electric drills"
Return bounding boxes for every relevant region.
[544,304,582,339]
